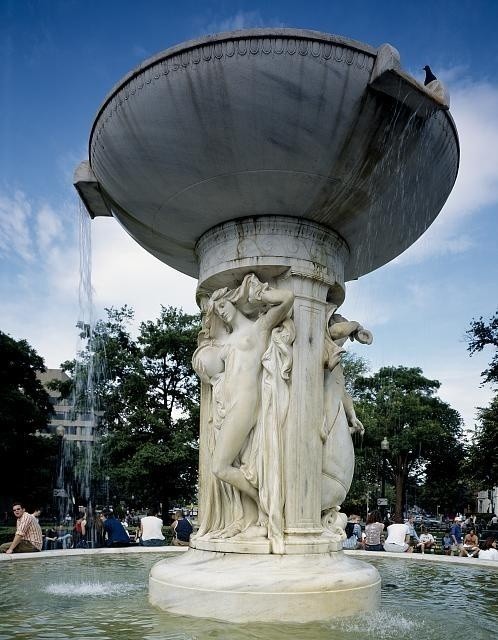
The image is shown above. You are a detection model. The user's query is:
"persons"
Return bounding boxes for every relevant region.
[343,510,498,560]
[192,284,294,539]
[0,502,192,554]
[320,314,365,442]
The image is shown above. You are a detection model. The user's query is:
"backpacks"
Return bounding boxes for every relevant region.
[345,523,359,539]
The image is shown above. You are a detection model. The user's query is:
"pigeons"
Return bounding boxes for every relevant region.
[421,64,437,87]
